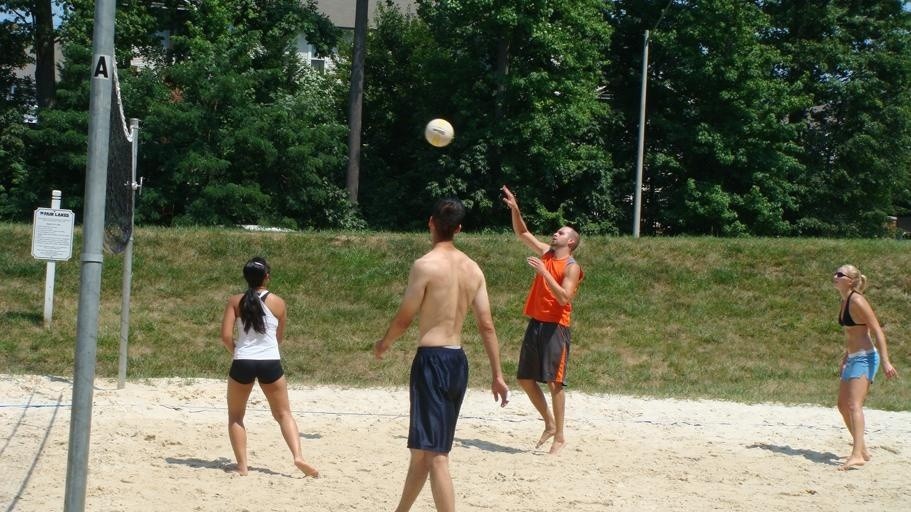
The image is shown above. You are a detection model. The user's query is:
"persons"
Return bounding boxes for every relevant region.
[367,197,511,512]
[218,254,324,481]
[829,262,900,472]
[497,179,587,458]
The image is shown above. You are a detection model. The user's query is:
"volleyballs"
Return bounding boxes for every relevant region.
[425,119,454,146]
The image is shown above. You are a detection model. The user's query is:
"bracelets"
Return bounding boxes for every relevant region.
[544,270,549,277]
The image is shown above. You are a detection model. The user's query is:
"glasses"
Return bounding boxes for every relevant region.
[834,272,852,278]
